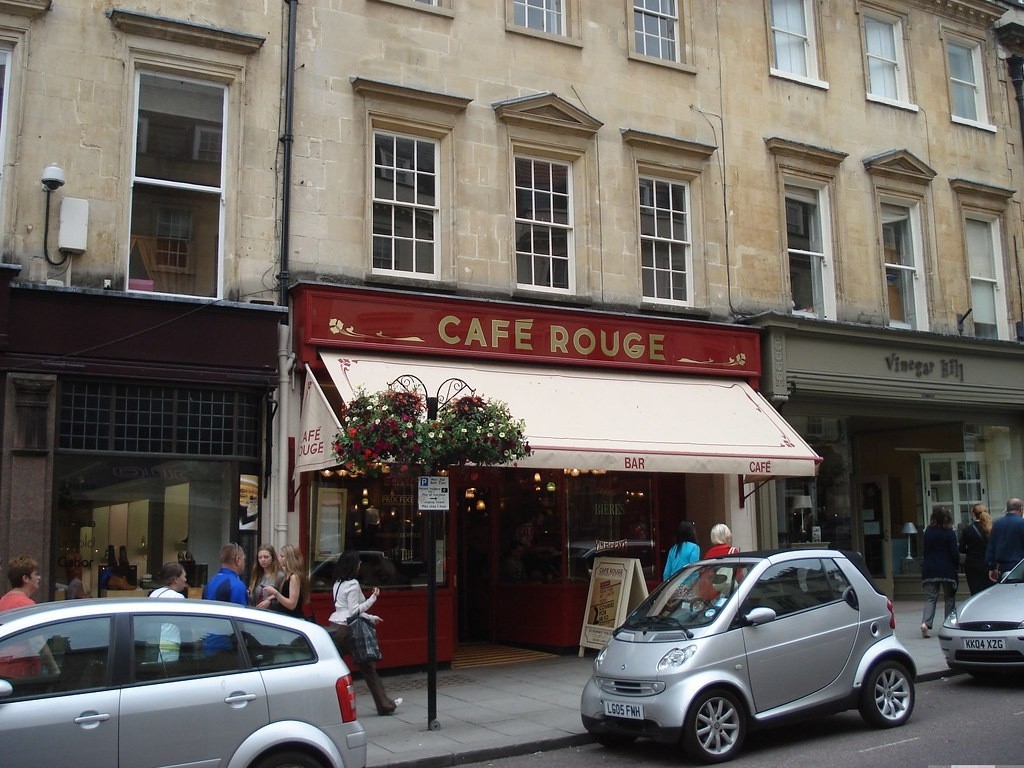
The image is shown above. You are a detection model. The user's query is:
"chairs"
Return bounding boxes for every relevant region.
[805,569,842,604]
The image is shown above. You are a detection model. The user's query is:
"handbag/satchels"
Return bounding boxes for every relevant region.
[712,547,739,598]
[345,608,382,664]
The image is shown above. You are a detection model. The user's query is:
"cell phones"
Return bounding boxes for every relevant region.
[260,584,265,589]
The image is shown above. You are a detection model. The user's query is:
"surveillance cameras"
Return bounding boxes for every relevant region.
[41,166,66,191]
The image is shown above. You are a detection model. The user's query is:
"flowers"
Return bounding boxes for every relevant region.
[330,376,535,478]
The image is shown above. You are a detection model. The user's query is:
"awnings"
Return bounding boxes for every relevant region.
[294,347,824,505]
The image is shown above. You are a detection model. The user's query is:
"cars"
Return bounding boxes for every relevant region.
[311,550,402,593]
[0,597,367,768]
[939,556,1024,678]
[580,546,918,765]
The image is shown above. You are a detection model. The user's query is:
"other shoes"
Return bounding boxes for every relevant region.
[921,622,931,638]
[394,697,403,707]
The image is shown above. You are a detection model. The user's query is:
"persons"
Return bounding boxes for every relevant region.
[147,561,188,662]
[920,505,959,638]
[261,544,308,617]
[960,504,994,595]
[0,556,61,678]
[663,521,701,603]
[500,510,548,582]
[202,542,249,657]
[365,504,381,530]
[327,551,403,715]
[699,523,745,603]
[985,497,1024,583]
[252,544,284,610]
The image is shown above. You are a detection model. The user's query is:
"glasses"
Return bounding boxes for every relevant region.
[233,542,239,550]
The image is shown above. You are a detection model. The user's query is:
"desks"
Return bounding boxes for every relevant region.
[778,542,830,549]
[101,587,204,599]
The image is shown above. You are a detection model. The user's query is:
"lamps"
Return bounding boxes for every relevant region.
[41,162,65,192]
[902,522,918,559]
[792,496,812,534]
